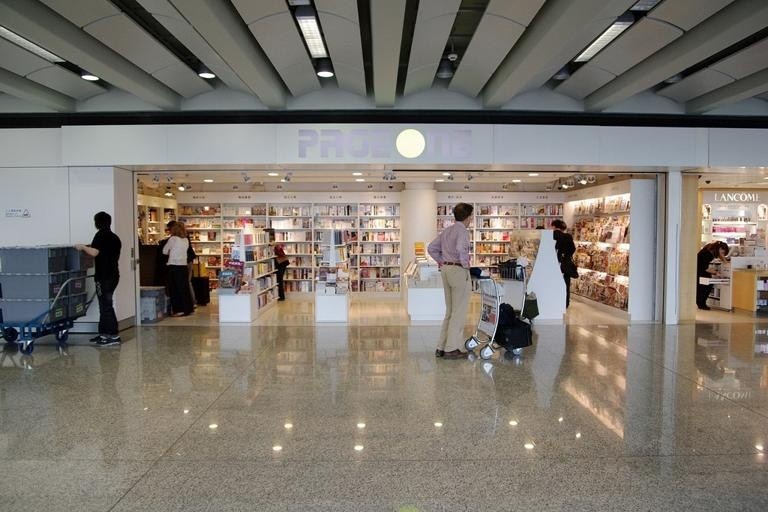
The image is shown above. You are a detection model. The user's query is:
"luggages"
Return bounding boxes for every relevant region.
[493,318,532,349]
[191,254,210,306]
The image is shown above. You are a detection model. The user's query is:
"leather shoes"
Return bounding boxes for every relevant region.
[436,349,467,359]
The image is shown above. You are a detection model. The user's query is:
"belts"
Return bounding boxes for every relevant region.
[442,261,462,266]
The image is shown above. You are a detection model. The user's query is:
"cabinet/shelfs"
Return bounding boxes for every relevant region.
[437,202,561,293]
[134,178,400,318]
[697,185,766,318]
[563,192,630,313]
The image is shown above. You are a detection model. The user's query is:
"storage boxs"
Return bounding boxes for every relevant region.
[0,242,97,333]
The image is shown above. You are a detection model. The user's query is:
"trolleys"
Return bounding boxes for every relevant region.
[466,268,527,360]
[1,344,83,389]
[0,274,102,356]
[464,350,525,448]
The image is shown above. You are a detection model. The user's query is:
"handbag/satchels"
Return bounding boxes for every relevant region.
[562,254,579,278]
[274,255,290,268]
[499,302,516,326]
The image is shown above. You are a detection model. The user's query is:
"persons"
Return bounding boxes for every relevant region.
[696,240,729,311]
[268,235,290,302]
[423,201,477,362]
[165,219,197,312]
[162,221,188,318]
[71,209,125,351]
[549,218,579,308]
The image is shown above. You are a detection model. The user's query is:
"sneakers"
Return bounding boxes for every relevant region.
[89,335,122,347]
